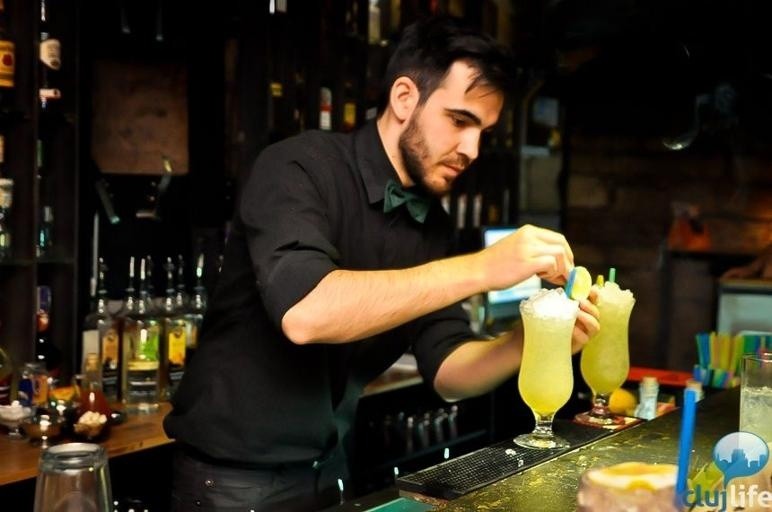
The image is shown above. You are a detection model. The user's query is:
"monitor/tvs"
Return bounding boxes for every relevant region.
[481,225,543,320]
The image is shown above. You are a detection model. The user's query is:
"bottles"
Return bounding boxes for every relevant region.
[39,0,67,102]
[79,252,210,415]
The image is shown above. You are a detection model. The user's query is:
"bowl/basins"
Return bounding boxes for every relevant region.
[0,404,108,441]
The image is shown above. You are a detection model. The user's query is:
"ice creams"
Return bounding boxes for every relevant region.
[513,268,592,450]
[575,268,635,426]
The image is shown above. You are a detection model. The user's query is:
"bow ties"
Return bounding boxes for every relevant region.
[382,178,428,224]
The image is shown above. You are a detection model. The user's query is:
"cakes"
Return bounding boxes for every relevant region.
[577,461,676,512]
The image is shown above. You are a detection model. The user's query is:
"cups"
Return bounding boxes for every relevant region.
[738,352,772,445]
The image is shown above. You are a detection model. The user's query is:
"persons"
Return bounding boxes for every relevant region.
[162,10,602,512]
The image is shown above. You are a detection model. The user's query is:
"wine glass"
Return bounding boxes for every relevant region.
[512,296,579,453]
[573,296,637,428]
